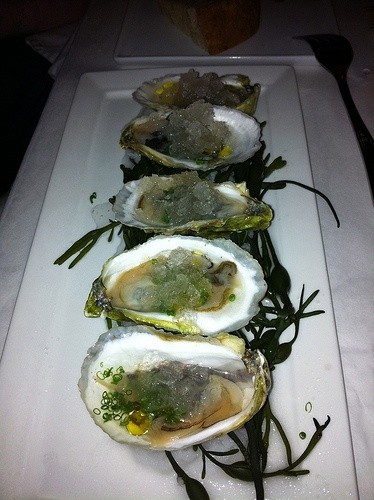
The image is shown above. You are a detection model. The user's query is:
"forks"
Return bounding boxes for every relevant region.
[293,33,374,207]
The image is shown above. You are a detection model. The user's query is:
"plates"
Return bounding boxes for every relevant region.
[113,0,341,69]
[0,64,362,500]
